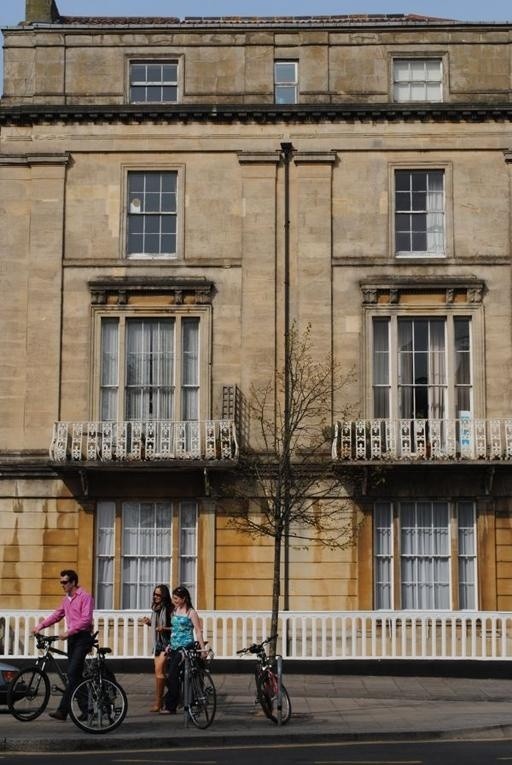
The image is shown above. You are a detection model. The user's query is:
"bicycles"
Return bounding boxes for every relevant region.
[7,631,95,723]
[67,631,128,735]
[237,633,291,725]
[165,641,218,730]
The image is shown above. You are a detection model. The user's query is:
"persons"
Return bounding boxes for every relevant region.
[155,585,209,716]
[141,582,178,713]
[30,568,95,723]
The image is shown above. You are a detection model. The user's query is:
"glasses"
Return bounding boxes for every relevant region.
[60,580,69,585]
[154,592,161,597]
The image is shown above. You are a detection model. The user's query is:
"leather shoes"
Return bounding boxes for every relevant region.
[77,713,89,722]
[49,713,67,721]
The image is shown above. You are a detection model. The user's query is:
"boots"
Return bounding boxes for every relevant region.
[150,678,166,712]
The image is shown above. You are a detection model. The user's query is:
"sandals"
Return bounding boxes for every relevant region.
[159,709,177,715]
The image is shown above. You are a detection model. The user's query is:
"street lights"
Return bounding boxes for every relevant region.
[269,141,294,659]
